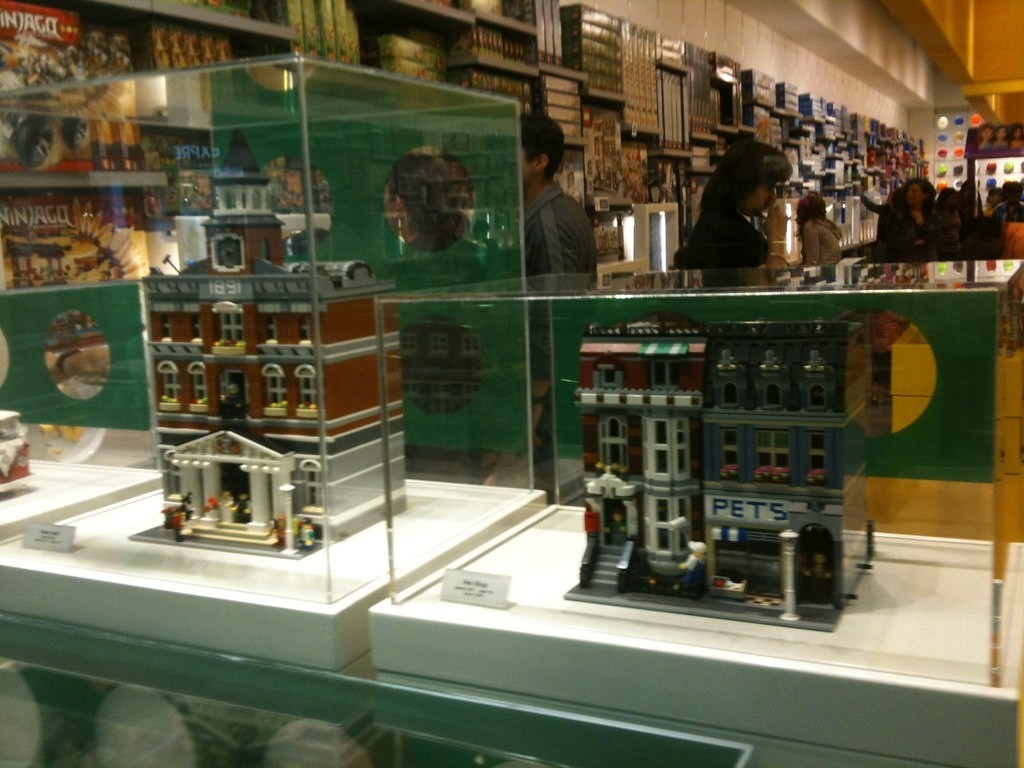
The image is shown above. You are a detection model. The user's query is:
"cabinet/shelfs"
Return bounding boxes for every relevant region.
[0,1,931,295]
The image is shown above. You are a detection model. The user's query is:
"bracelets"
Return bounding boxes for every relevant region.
[533,396,545,405]
[768,240,785,245]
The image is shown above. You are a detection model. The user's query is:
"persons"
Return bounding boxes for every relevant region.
[861,178,941,262]
[795,191,842,269]
[519,110,596,505]
[674,139,789,288]
[961,232,1001,260]
[988,179,1024,222]
[998,221,1024,336]
[381,157,508,483]
[935,179,983,261]
